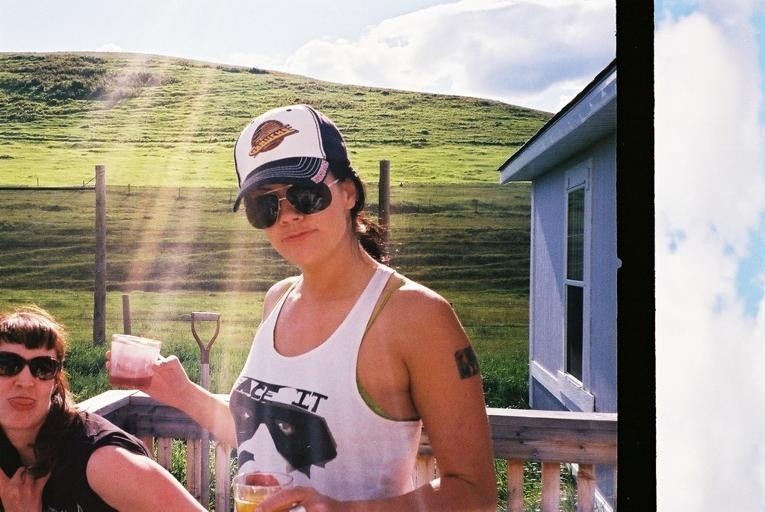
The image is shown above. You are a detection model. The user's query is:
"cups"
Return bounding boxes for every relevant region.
[232,470,294,512]
[109,334,162,390]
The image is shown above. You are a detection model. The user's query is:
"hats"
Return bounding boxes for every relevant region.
[231,103,347,213]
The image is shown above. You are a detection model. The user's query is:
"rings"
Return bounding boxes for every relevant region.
[288,503,307,512]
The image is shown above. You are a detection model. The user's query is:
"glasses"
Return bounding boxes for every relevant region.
[0,351,61,380]
[246,178,340,229]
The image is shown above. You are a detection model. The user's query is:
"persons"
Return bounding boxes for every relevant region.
[1,302,210,512]
[104,102,499,512]
